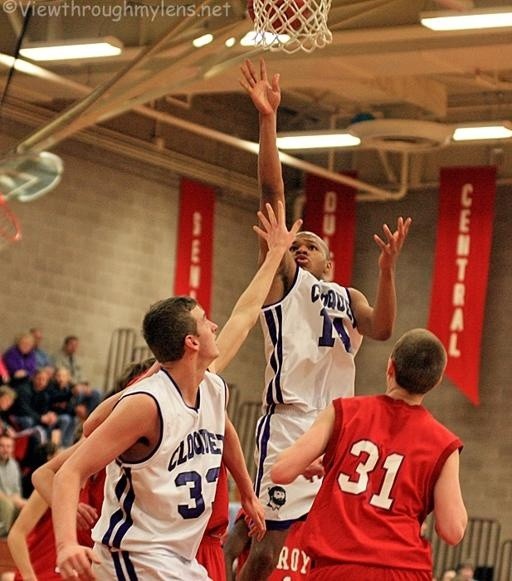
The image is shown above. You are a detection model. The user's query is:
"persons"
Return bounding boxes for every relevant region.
[236,51,414,581]
[50,295,265,581]
[0,326,231,580]
[263,329,469,581]
[226,503,265,581]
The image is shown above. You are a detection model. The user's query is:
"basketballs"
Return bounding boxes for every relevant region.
[248,0,310,34]
